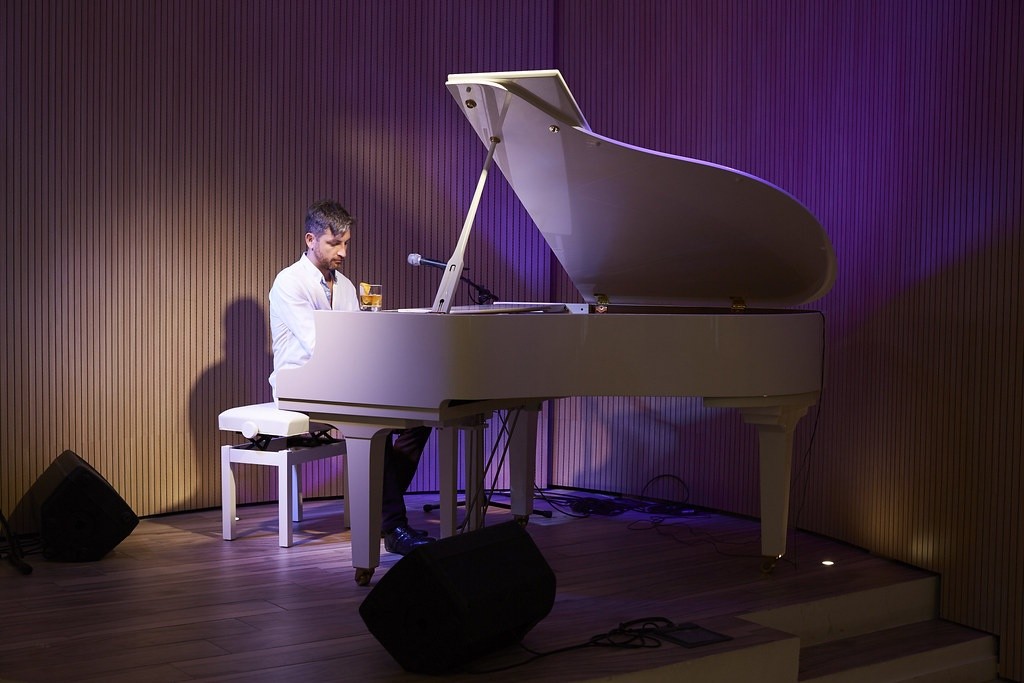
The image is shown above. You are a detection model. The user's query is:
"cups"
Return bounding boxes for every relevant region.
[360,283,382,311]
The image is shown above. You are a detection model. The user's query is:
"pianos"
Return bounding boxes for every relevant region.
[275,68,838,587]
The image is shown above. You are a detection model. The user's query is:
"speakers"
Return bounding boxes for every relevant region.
[356,521,557,677]
[33,450,140,563]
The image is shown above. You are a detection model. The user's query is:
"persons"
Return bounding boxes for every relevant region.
[268,202,437,553]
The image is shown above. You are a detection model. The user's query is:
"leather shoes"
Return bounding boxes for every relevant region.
[381,524,429,539]
[384,525,436,556]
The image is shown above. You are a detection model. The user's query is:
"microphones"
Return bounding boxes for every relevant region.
[407,254,447,271]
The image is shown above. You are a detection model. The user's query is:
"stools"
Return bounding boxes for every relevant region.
[218,401,351,549]
